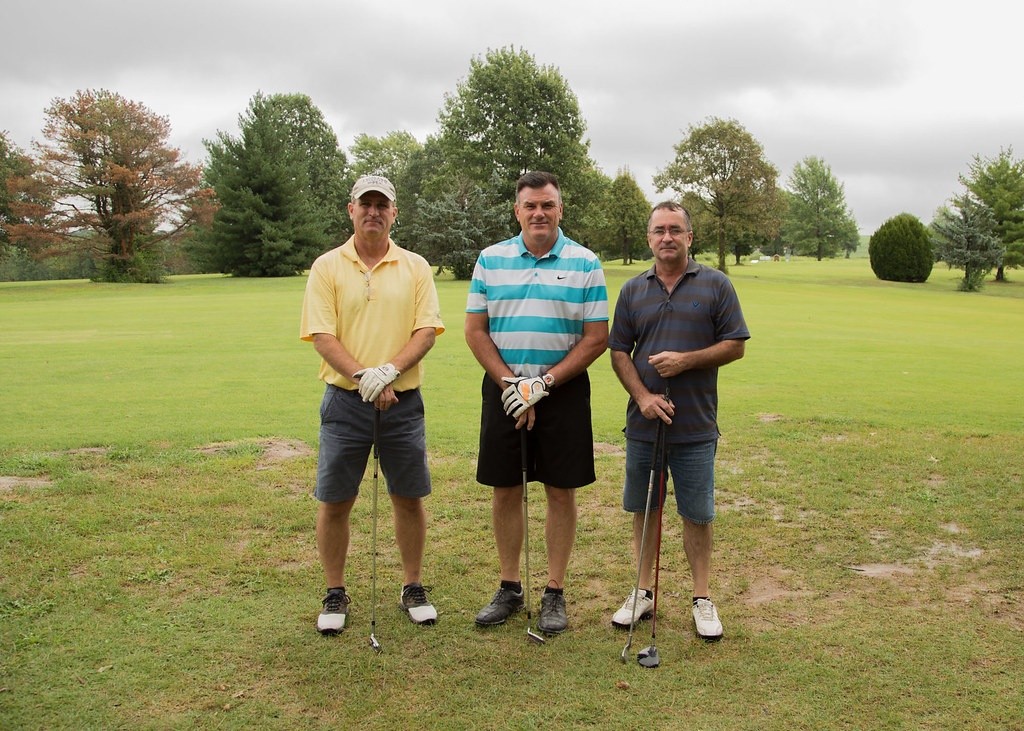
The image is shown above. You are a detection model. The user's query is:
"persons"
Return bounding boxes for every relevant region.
[607,203,753,640]
[300,176,448,635]
[466,171,611,636]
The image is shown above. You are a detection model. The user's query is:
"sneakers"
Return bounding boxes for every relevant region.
[317,588,348,634]
[538,593,568,634]
[474,588,525,626]
[691,599,723,641]
[400,582,438,625]
[612,588,654,628]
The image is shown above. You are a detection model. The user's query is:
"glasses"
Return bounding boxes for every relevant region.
[649,230,689,236]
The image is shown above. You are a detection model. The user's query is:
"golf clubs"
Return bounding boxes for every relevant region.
[620,376,673,670]
[368,406,382,652]
[518,420,547,645]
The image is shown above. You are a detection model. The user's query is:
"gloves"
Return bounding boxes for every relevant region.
[500,375,550,420]
[351,362,401,403]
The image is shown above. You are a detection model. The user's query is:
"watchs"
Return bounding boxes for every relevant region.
[541,372,557,393]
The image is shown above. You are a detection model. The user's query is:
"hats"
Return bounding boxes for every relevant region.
[349,176,396,201]
